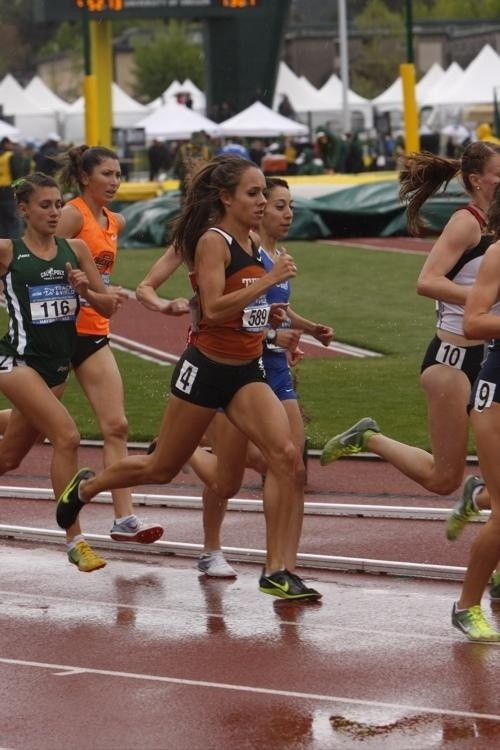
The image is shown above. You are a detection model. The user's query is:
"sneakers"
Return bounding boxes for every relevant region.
[56,468,163,572]
[446,475,485,540]
[320,417,381,467]
[452,572,500,642]
[195,550,237,577]
[259,567,321,600]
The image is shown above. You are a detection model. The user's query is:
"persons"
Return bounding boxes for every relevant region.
[0,179,119,573]
[1,124,500,194]
[1,147,164,544]
[322,141,499,539]
[451,180,499,640]
[56,154,335,600]
